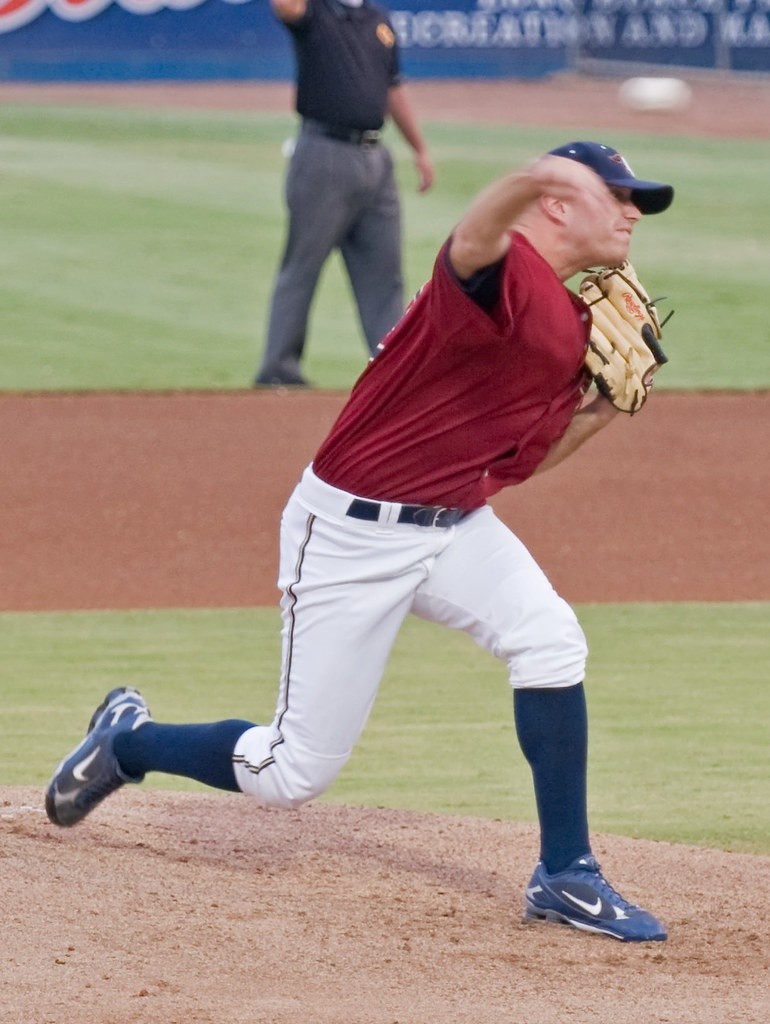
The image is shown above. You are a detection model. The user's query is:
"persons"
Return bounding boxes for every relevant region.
[43,141,675,943]
[257,0,436,387]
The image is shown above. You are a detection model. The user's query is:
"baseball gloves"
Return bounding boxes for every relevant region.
[579,258,670,414]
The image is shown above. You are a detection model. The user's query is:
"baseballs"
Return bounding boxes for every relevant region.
[621,77,693,117]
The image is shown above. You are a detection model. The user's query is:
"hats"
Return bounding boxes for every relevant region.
[547,140,675,215]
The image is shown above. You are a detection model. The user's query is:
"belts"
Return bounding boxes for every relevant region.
[346,499,470,527]
[326,126,383,145]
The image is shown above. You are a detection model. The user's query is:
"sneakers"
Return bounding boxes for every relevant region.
[524,853,668,942]
[45,686,155,828]
[255,373,310,388]
